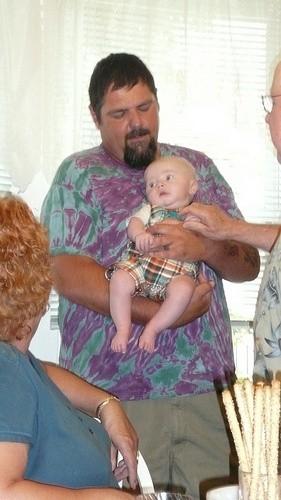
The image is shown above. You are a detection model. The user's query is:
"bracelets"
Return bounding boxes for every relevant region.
[95,395,120,419]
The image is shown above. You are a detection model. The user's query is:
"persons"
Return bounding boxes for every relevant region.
[105,155,201,352]
[39,53,259,500]
[179,62,280,383]
[0,194,138,500]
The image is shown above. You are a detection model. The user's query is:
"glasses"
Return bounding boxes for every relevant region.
[261,94,281,114]
